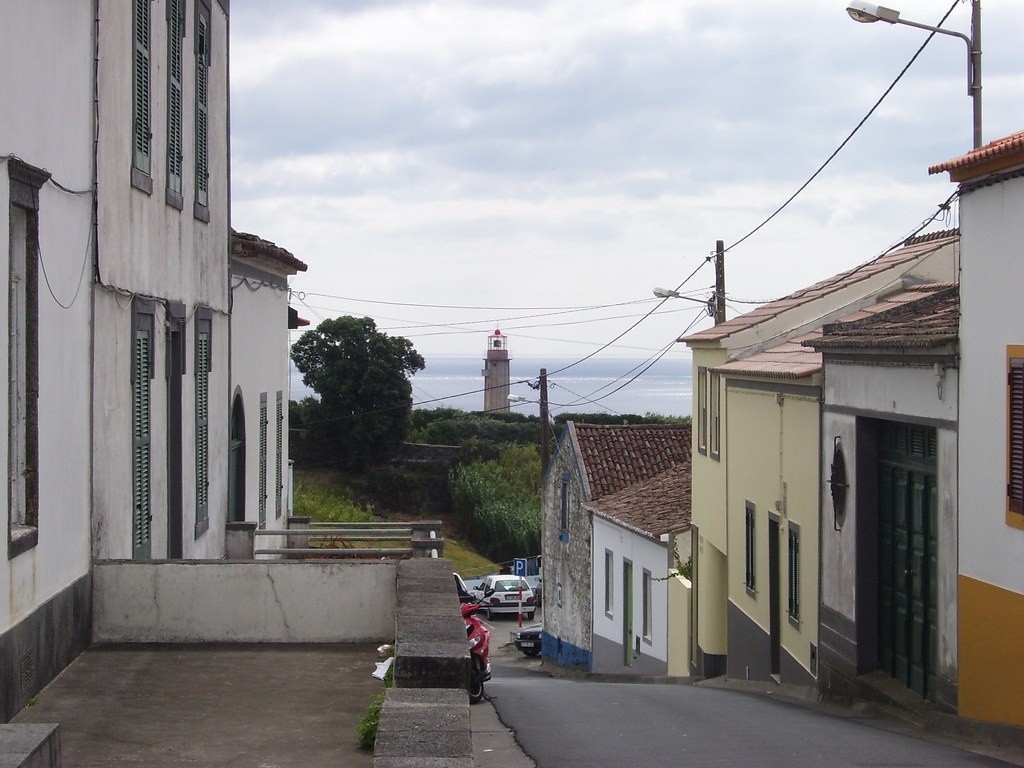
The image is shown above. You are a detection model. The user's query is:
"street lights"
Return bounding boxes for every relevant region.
[507,396,548,481]
[653,287,726,326]
[847,1,983,148]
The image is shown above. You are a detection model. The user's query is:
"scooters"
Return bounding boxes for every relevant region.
[460,588,496,703]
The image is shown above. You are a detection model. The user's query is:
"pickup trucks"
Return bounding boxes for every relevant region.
[452,573,476,618]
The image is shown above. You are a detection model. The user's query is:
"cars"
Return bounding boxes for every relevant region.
[535,577,542,607]
[514,619,543,657]
[473,575,536,619]
